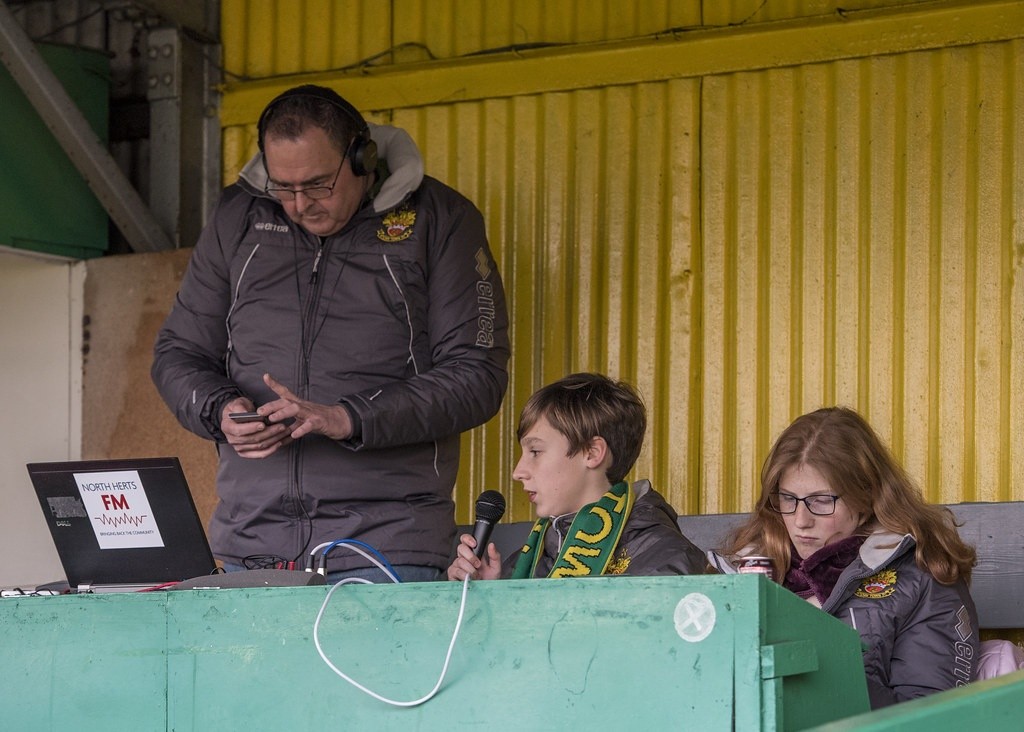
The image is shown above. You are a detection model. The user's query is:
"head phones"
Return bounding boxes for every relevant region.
[256,92,379,175]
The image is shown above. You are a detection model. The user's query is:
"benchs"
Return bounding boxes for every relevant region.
[452,501,1024,630]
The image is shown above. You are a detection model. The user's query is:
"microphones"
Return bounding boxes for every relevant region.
[469,489,505,561]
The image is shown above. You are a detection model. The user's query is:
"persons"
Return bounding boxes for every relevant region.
[707,406,979,711]
[151,84,510,585]
[449,373,707,581]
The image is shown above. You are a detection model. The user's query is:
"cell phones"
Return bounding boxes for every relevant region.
[229,412,273,428]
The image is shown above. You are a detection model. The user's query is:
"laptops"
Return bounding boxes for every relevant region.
[26,458,219,591]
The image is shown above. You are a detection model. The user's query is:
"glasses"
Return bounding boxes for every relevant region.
[265,135,355,200]
[768,492,840,516]
